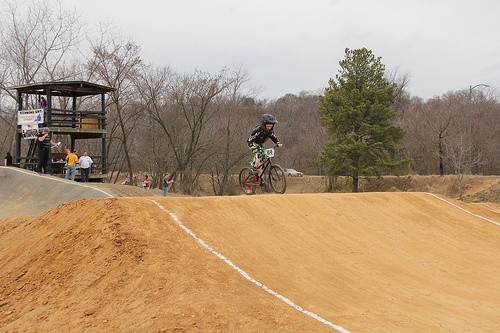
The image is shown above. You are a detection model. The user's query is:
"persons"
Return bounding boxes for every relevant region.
[79,151,93,183]
[5,152,12,167]
[36,127,59,175]
[247,114,283,187]
[66,149,80,181]
[143,175,150,190]
[163,173,174,196]
[39,97,48,125]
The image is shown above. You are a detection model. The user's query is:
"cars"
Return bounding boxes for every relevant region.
[274,168,304,178]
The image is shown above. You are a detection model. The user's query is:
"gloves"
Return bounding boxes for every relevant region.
[277,142,283,148]
[251,146,258,154]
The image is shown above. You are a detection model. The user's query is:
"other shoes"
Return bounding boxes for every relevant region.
[250,162,258,171]
[261,179,266,184]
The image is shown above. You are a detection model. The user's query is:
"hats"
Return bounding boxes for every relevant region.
[44,127,50,132]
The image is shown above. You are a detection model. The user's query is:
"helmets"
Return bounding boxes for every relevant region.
[260,114,277,134]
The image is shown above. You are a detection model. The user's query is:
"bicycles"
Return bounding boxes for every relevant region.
[239,142,287,196]
